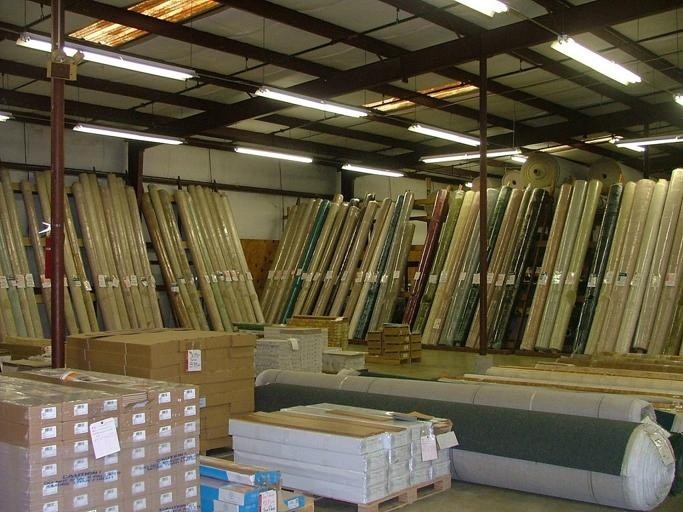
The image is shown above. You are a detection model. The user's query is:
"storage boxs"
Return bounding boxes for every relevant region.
[65,330,256,440]
[229,402,454,506]
[199,455,315,512]
[0,335,197,512]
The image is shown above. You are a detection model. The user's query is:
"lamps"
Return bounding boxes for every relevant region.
[72,123,187,148]
[454,1,508,18]
[341,162,403,177]
[16,32,200,82]
[2,111,15,124]
[255,86,368,120]
[408,123,482,147]
[551,38,643,87]
[609,134,683,152]
[233,142,312,164]
[673,92,683,106]
[418,147,527,164]
